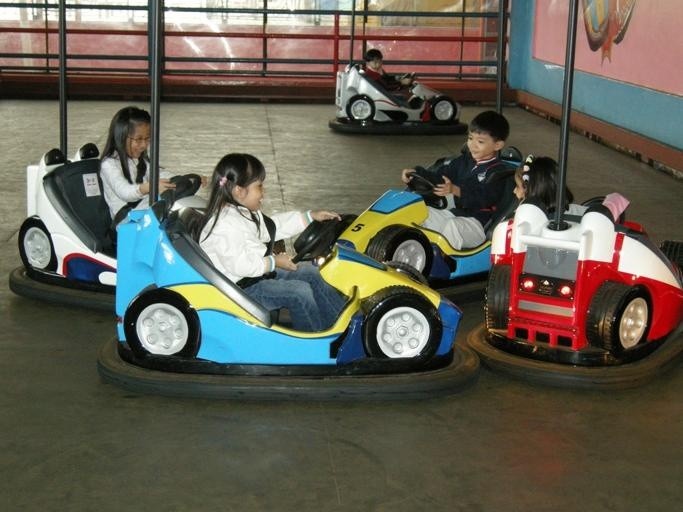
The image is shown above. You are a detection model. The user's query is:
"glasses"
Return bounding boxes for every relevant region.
[124,136,150,143]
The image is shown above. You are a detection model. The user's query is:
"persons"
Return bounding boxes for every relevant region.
[98,105,207,234]
[401,110,517,251]
[198,153,347,333]
[513,156,630,224]
[364,48,413,103]
